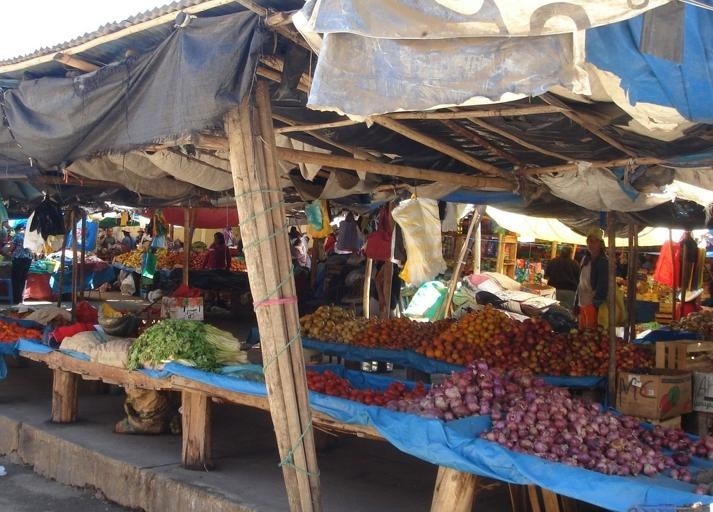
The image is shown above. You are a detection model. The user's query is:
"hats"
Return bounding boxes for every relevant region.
[121,226,131,233]
[136,227,145,233]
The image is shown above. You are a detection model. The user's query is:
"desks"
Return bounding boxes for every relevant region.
[177,353,492,511]
[433,415,712,510]
[16,320,178,425]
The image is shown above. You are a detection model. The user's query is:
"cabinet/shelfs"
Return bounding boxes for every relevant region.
[439,232,712,321]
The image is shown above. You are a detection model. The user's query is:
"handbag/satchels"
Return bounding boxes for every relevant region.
[597,283,625,330]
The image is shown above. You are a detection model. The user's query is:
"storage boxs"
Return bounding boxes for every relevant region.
[693,370,712,414]
[614,367,695,420]
[650,417,681,431]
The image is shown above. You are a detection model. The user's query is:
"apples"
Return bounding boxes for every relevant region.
[349,302,653,377]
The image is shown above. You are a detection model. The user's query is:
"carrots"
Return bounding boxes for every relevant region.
[0,320,43,343]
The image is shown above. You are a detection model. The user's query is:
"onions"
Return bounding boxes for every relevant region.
[387,358,713,496]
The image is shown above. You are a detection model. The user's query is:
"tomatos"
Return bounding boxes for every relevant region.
[306,369,427,407]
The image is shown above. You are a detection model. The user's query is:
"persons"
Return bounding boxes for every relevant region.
[569,227,608,332]
[202,227,238,272]
[542,244,580,306]
[1,218,35,304]
[288,226,312,268]
[103,226,144,250]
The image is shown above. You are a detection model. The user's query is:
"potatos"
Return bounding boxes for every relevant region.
[670,309,713,336]
[300,303,381,345]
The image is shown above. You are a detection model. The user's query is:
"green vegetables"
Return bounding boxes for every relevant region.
[127,318,248,373]
